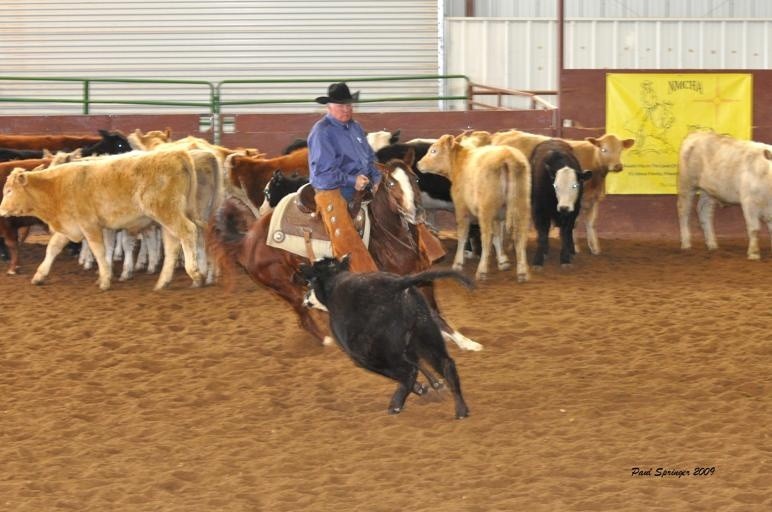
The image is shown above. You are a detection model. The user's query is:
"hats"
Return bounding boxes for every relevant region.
[317,82,362,107]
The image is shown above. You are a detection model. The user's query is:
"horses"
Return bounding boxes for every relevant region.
[204,147,484,353]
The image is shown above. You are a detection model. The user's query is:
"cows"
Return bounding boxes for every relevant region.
[0,126,310,291]
[677,131,772,260]
[529,139,593,273]
[417,134,533,283]
[455,130,491,259]
[363,130,451,259]
[289,252,478,420]
[491,129,634,255]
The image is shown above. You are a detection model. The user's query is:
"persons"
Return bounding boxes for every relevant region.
[306,81,448,277]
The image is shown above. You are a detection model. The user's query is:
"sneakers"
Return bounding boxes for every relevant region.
[432,255,445,264]
